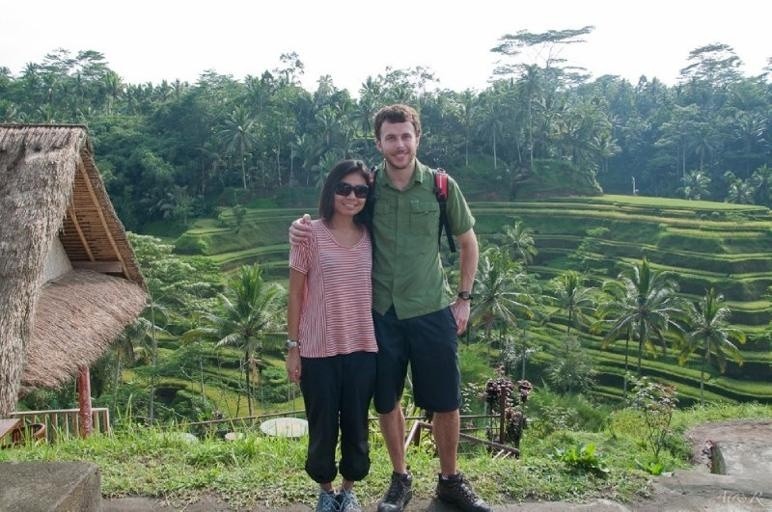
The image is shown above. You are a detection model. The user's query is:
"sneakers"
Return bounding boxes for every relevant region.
[377,471,413,511]
[437,473,491,512]
[337,486,362,512]
[316,488,337,512]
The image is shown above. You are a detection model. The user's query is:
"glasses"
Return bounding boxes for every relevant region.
[334,181,369,199]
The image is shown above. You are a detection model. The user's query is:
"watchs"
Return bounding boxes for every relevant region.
[284,338,299,352]
[458,290,474,302]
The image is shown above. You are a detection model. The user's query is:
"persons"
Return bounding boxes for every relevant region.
[283,154,381,510]
[288,101,494,510]
